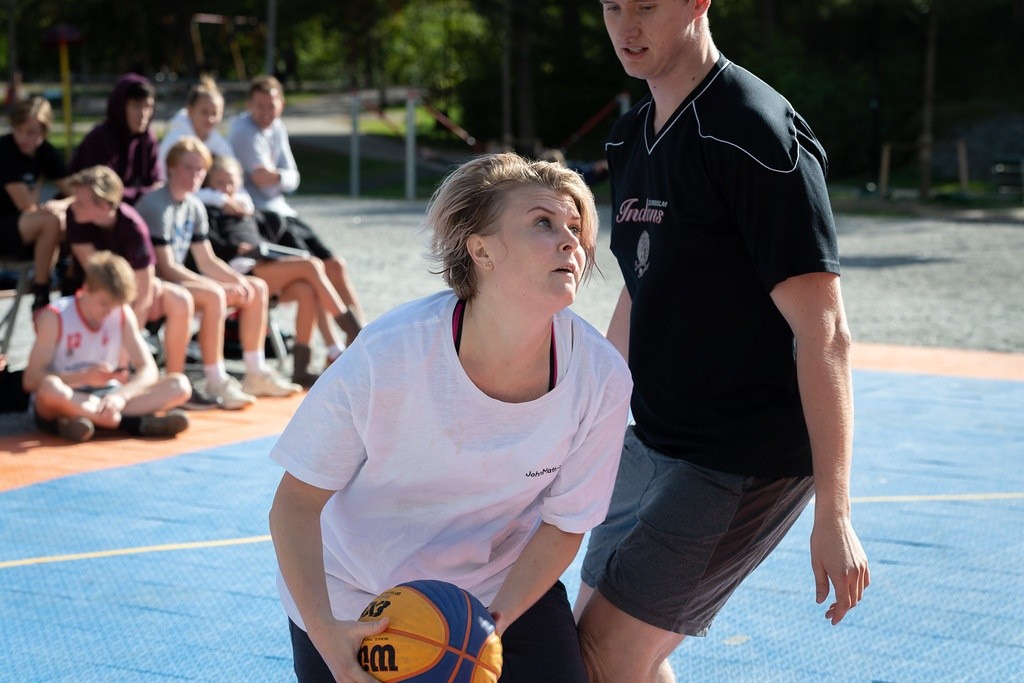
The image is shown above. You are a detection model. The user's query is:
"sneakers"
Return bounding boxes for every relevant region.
[242,369,302,396]
[205,375,257,409]
[322,352,342,372]
[180,387,217,409]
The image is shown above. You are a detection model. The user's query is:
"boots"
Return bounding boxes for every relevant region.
[291,345,318,388]
[335,309,361,348]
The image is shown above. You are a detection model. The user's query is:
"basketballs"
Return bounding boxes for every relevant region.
[354,578,504,683]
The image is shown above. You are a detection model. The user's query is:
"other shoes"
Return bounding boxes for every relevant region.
[140,414,188,438]
[31,277,53,313]
[58,415,95,441]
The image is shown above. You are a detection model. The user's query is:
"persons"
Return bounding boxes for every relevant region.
[0,74,361,442]
[574,0,870,683]
[267,154,635,682]
